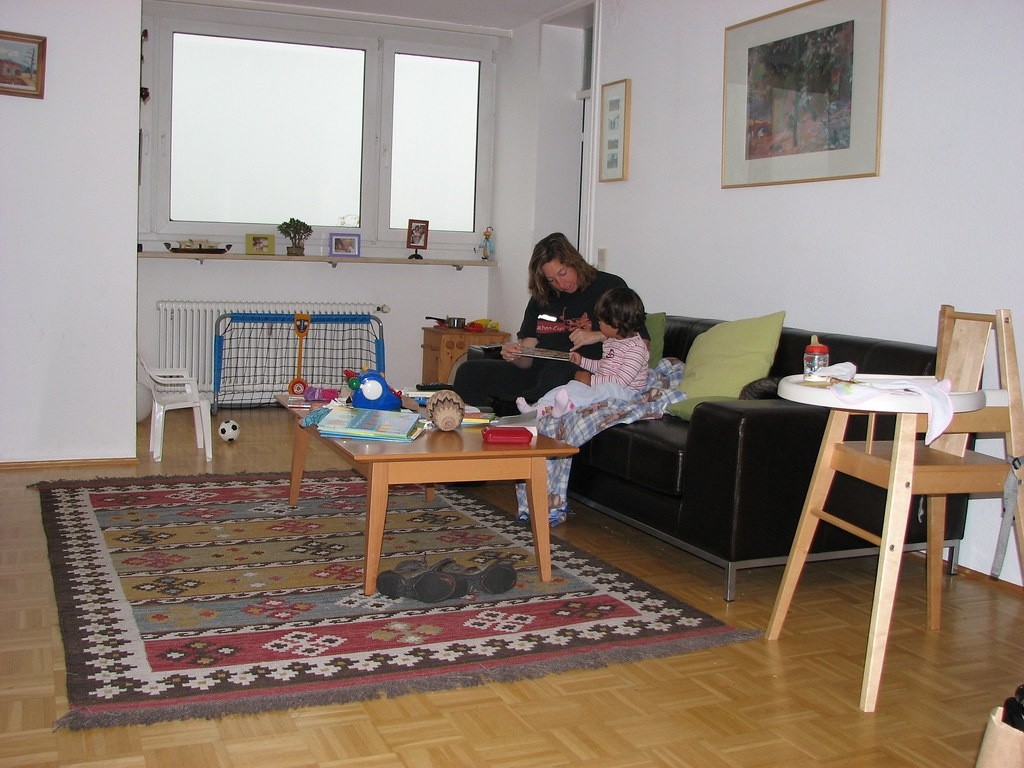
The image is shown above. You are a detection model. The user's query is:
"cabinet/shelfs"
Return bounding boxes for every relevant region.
[421,326,511,385]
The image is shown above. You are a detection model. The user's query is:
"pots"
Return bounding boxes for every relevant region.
[425,316,465,328]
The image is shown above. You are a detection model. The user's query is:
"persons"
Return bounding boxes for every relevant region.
[453,232,628,405]
[516,286,650,418]
[411,225,424,246]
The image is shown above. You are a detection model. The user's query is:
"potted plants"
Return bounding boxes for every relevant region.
[277,218,313,256]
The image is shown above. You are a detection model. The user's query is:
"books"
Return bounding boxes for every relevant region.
[406,388,436,397]
[460,413,499,426]
[288,400,311,407]
[317,407,426,443]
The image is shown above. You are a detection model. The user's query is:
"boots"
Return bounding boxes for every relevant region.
[375,559,456,605]
[430,557,519,597]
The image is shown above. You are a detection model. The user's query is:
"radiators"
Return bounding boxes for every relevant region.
[155,299,377,394]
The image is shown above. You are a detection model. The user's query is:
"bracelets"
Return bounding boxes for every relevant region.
[516,343,524,347]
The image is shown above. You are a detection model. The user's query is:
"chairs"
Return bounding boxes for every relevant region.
[765,303,1024,712]
[136,349,213,463]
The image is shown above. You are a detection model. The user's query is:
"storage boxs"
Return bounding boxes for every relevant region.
[974,706,1024,768]
[496,409,540,436]
[481,426,533,444]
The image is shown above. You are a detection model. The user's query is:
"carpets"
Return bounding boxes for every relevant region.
[26,469,766,734]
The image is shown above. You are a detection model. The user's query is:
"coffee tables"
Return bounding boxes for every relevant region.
[273,394,581,596]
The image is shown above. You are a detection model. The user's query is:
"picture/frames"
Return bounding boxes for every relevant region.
[721,0,887,190]
[0,31,47,100]
[599,78,631,183]
[246,234,275,255]
[407,219,429,250]
[328,233,361,258]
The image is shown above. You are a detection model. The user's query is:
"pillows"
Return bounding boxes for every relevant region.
[738,377,782,400]
[665,395,739,421]
[644,312,667,369]
[678,310,786,398]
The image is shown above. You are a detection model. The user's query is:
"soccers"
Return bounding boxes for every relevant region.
[217,419,241,442]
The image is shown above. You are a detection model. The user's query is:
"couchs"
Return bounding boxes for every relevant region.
[469,312,984,602]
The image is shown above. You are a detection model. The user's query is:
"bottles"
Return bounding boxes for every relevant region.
[804,336,829,381]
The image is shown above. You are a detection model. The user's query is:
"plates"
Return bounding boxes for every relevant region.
[464,327,484,333]
